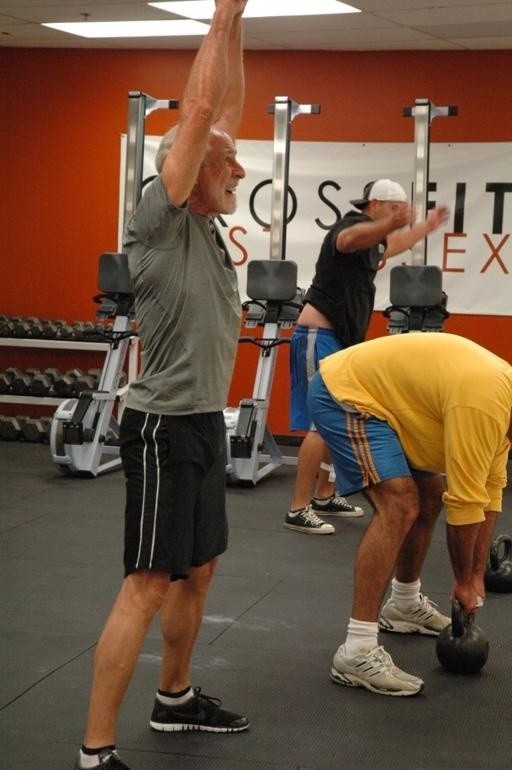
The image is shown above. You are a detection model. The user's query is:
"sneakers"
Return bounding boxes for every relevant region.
[73,748,130,770]
[149,686,249,733]
[377,593,453,636]
[330,645,425,698]
[309,492,364,517]
[284,503,335,535]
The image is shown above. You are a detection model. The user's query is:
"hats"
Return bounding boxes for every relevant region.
[349,178,409,209]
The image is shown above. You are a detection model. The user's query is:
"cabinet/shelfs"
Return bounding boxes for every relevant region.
[0,335,138,426]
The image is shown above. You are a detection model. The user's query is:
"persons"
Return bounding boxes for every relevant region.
[80,1,248,769]
[282,178,451,536]
[309,331,512,698]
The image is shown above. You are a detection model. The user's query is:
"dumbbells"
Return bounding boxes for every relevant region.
[1,367,101,396]
[2,315,112,342]
[1,414,51,443]
